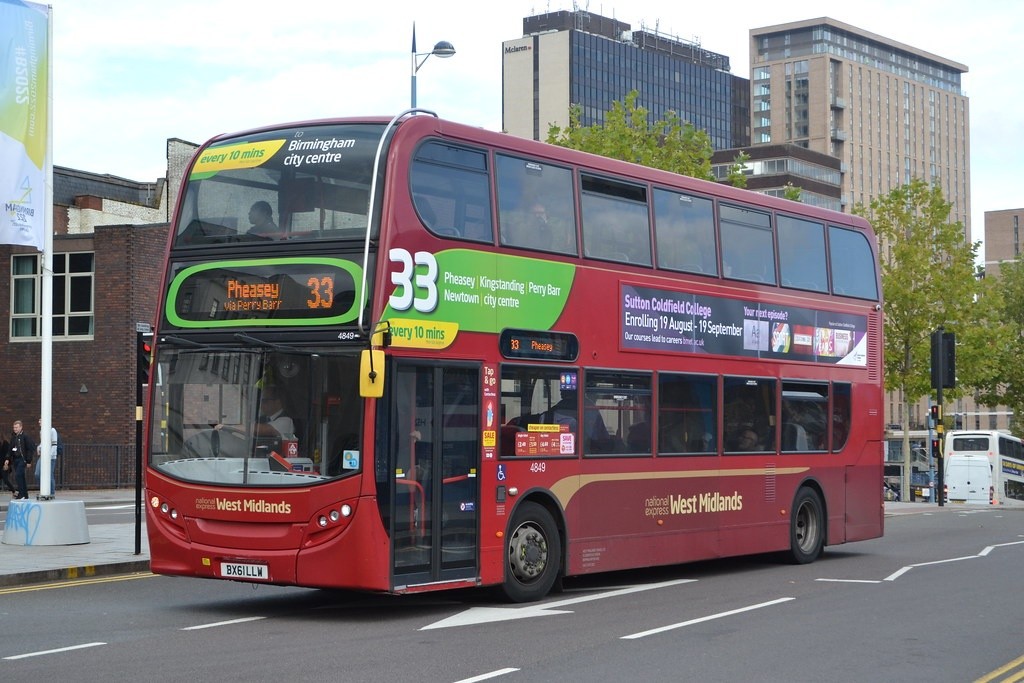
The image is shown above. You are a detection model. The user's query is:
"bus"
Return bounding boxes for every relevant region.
[143,109,885,603]
[943,428,1024,507]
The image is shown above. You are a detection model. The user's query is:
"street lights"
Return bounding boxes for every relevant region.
[412,21,456,116]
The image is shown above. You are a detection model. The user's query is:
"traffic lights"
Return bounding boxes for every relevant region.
[931,405,941,420]
[932,439,942,458]
[142,332,162,387]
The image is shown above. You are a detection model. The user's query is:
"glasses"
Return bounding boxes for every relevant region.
[258,398,274,404]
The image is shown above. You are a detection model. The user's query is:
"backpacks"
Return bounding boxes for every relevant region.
[57,436,64,455]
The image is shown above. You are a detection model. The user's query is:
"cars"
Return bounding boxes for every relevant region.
[883,480,899,501]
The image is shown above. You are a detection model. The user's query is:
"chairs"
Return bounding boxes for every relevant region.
[434,226,846,296]
[627,420,809,453]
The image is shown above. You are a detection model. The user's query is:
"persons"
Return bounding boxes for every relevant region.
[215,386,295,457]
[37,417,57,497]
[246,201,279,234]
[886,483,900,501]
[526,202,550,235]
[0,421,34,499]
[549,391,847,453]
